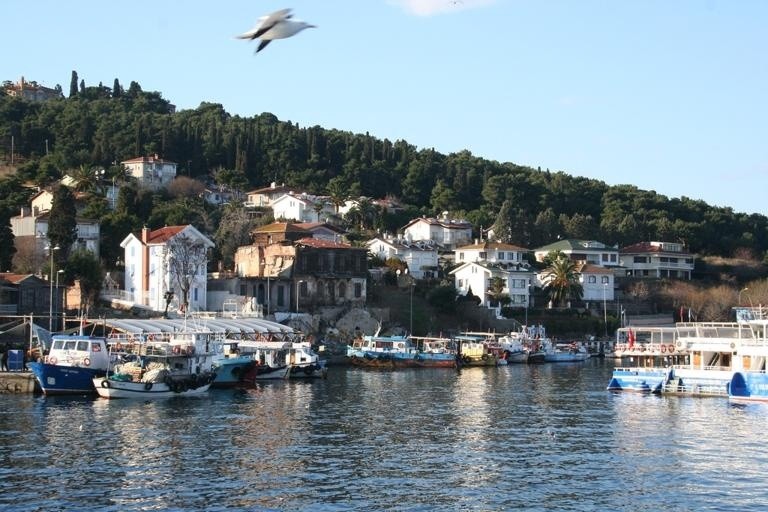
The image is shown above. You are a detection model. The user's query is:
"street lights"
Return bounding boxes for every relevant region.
[739,287,749,306]
[602,283,608,323]
[45,246,65,331]
[296,281,303,314]
[408,278,416,336]
[522,299,529,326]
[261,260,275,315]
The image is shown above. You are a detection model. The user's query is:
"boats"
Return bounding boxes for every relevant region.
[607,306,768,403]
[347,325,590,368]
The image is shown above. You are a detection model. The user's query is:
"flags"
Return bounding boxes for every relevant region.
[628,328,635,347]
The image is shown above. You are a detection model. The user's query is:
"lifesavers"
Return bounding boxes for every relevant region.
[84,358,91,367]
[48,357,57,365]
[398,343,404,348]
[738,310,752,325]
[618,344,675,353]
[173,346,181,355]
[185,345,195,356]
[115,342,123,353]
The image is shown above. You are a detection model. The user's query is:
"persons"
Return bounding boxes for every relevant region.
[115,343,124,353]
[568,340,579,354]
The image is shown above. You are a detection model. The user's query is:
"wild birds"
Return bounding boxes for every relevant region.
[233,9,318,53]
